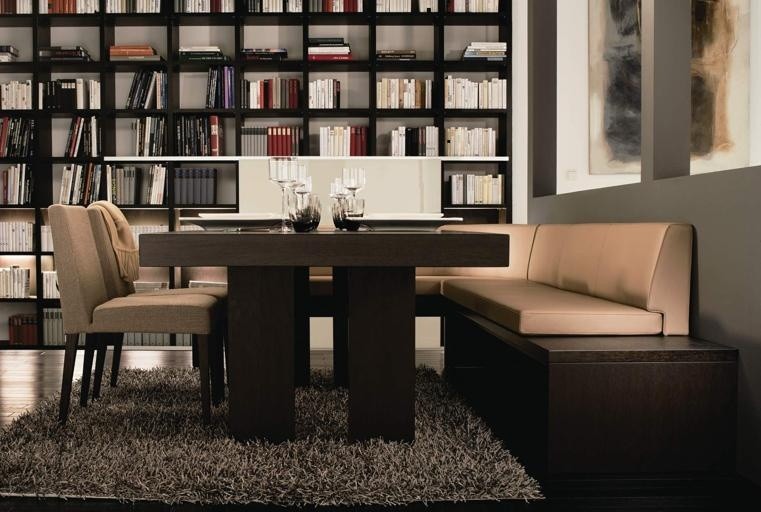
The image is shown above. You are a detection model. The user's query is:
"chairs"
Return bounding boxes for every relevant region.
[87,200,227,406]
[48,205,219,431]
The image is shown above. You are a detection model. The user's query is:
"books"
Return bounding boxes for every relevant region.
[451,173,505,205]
[1,45,34,206]
[1,0,235,13]
[241,0,499,13]
[307,37,368,156]
[0,206,230,350]
[445,41,508,157]
[107,45,166,206]
[377,49,439,156]
[39,45,101,207]
[242,47,305,156]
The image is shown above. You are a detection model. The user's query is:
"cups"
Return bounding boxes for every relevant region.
[330,198,365,231]
[289,194,322,232]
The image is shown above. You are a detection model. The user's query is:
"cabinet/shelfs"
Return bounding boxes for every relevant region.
[1,0,519,349]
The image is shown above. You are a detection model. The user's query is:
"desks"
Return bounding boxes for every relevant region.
[133,226,510,450]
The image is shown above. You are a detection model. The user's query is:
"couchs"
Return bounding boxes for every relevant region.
[296,219,741,507]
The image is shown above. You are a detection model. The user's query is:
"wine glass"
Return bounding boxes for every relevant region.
[268,155,312,232]
[329,167,364,200]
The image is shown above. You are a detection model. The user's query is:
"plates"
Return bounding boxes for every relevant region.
[176,216,290,232]
[345,215,464,231]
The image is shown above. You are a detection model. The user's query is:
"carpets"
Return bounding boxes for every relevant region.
[0,360,545,508]
